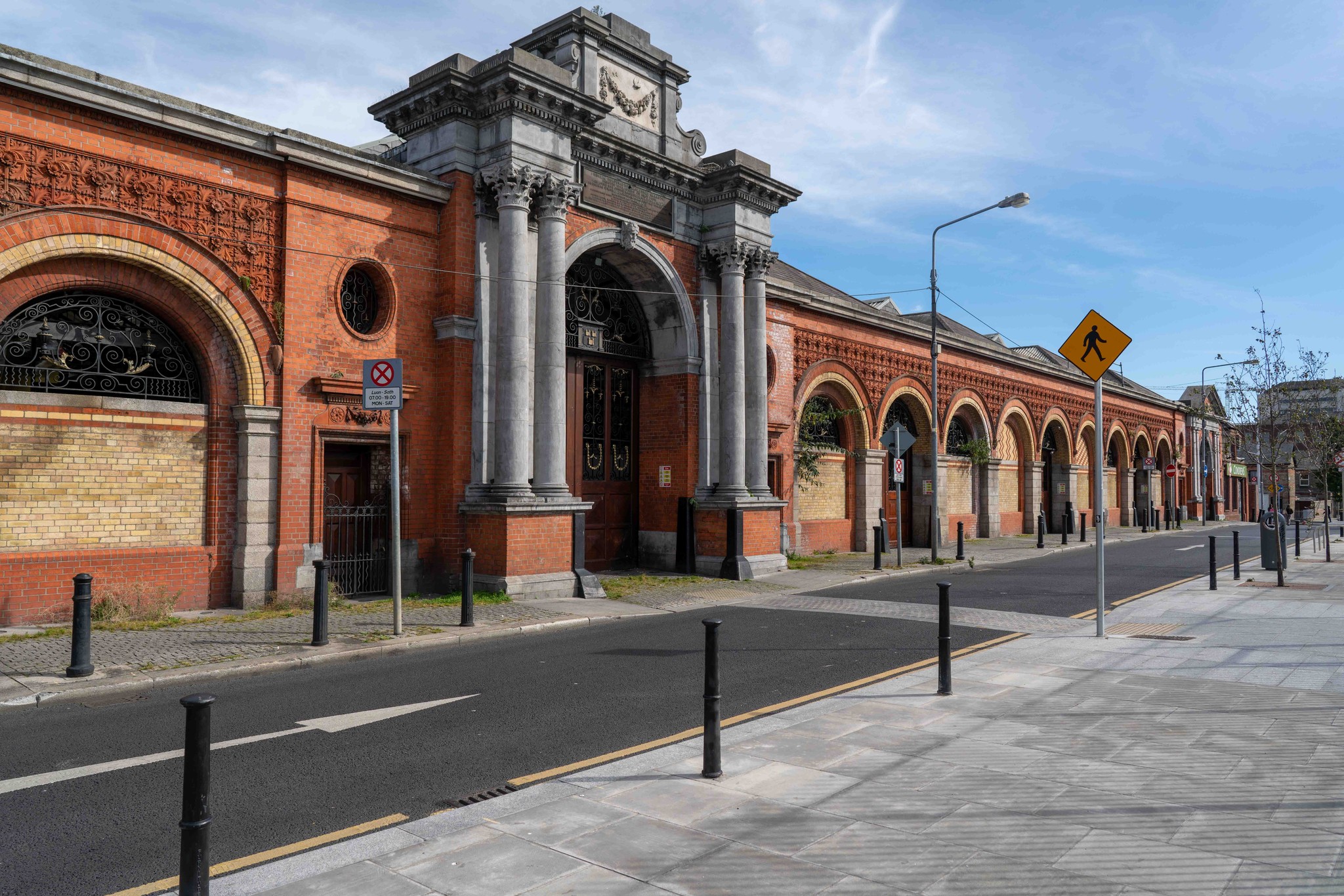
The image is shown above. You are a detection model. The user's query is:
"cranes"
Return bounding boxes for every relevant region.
[1142,377,1266,423]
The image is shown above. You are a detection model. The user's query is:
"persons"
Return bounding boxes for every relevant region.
[1322,506,1332,523]
[1268,504,1274,525]
[1286,504,1293,526]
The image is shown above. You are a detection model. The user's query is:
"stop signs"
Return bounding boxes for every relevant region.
[1166,464,1176,478]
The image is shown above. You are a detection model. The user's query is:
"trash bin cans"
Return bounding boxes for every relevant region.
[1260,511,1287,570]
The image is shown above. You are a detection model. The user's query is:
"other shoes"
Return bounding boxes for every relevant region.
[1287,524,1290,526]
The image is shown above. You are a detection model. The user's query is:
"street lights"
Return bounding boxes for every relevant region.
[932,192,1030,564]
[1202,360,1260,526]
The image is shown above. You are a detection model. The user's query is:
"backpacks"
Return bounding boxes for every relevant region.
[1282,508,1287,516]
[1270,508,1274,512]
